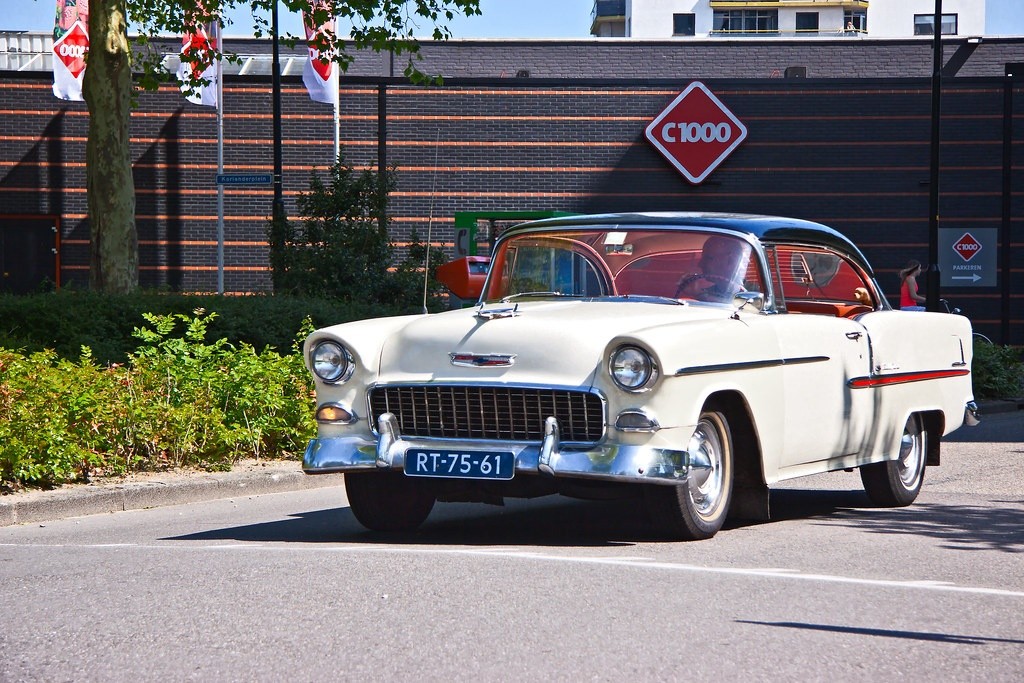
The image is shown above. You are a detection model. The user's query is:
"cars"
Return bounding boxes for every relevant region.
[302,211,984,542]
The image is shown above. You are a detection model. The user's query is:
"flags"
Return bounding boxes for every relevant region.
[301,1,336,104]
[178,0,218,107]
[52,0,89,101]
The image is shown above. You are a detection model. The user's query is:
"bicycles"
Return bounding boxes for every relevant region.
[939,298,994,347]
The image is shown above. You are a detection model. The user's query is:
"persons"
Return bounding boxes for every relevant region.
[694,235,761,303]
[847,22,855,32]
[899,260,926,311]
